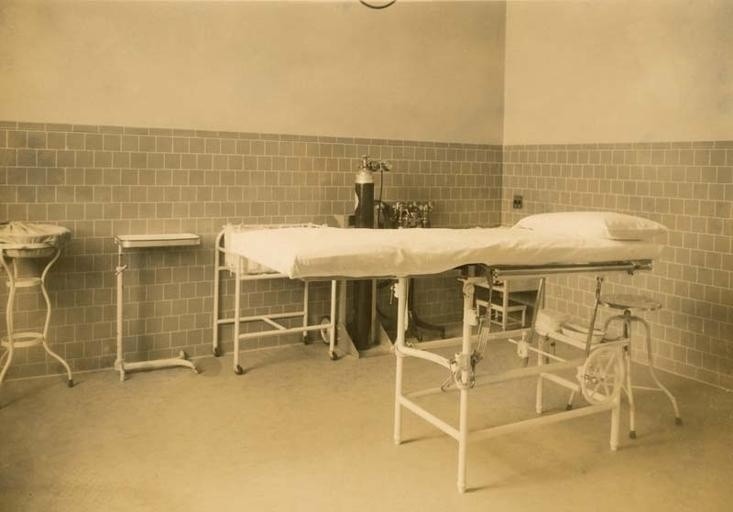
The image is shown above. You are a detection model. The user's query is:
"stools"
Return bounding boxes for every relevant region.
[570,294,680,438]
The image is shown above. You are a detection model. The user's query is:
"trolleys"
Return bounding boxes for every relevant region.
[212,225,338,375]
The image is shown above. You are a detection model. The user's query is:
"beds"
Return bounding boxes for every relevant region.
[227,230,663,494]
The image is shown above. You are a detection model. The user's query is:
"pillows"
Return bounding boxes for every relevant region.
[516,212,671,243]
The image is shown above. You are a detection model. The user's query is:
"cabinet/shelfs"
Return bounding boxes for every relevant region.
[1,244,74,387]
[112,233,201,381]
[471,266,545,332]
